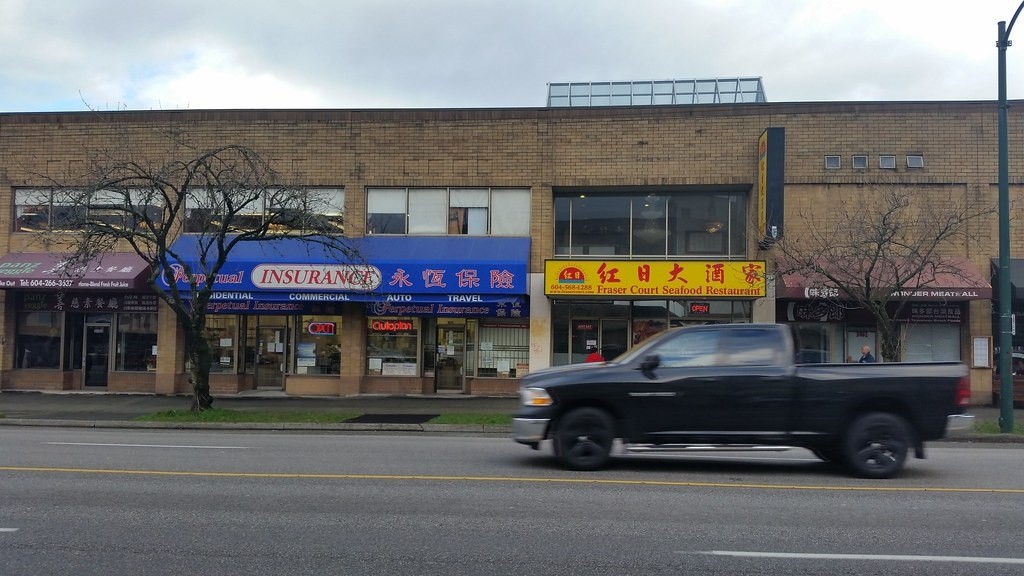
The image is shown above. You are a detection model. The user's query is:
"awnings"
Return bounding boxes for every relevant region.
[776,256,992,300]
[994,258,1024,296]
[1,252,158,292]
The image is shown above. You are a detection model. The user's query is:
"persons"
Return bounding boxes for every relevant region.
[858,345,875,363]
[1016,359,1023,375]
[585,346,605,363]
[320,344,333,373]
[86,343,95,376]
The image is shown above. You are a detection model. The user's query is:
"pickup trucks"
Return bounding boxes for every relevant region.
[511,317,976,478]
